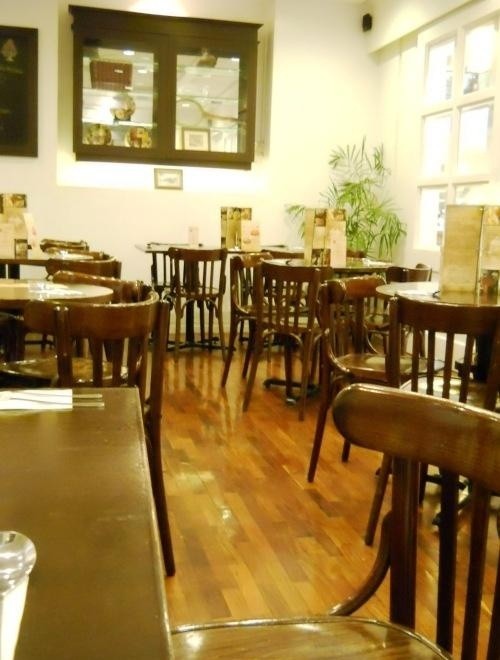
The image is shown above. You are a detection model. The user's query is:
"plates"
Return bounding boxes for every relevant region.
[369,262,386,266]
[86,123,112,145]
[124,126,153,149]
[109,93,135,120]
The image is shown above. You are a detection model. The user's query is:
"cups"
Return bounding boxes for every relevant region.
[15,237,29,259]
[362,257,370,266]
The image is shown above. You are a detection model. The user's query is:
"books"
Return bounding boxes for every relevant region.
[0,193,29,258]
[220,206,252,250]
[442,202,500,294]
[304,205,346,264]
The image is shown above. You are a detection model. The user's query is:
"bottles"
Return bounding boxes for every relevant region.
[1,528,36,660]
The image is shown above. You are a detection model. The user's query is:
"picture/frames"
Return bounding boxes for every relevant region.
[181,126,211,151]
[0,24,38,158]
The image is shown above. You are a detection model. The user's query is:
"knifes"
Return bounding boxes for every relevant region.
[5,389,106,407]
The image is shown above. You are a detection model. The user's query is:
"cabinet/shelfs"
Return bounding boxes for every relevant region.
[174,64,247,153]
[82,59,154,148]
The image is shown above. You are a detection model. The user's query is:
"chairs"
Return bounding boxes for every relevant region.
[0,239,500,660]
